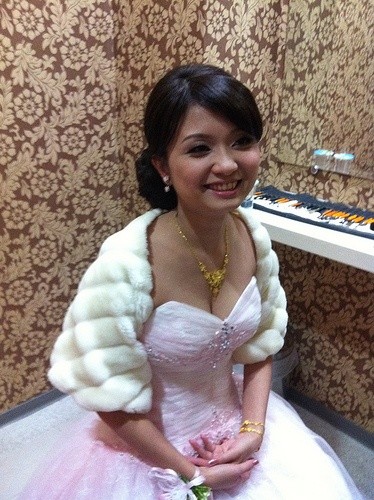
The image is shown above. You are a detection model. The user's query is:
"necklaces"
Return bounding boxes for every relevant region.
[173,210,231,303]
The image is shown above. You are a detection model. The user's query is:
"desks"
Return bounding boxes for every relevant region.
[233,185,374,278]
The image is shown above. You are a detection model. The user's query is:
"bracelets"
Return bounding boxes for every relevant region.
[238,420,265,438]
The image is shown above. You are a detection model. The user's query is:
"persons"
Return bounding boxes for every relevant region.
[19,64,369,500]
